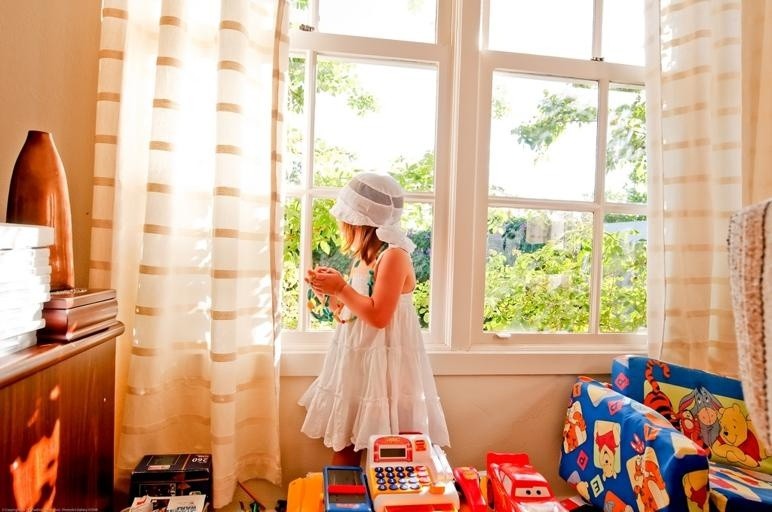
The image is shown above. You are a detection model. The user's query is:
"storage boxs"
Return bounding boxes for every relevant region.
[131,453,212,512]
[39,289,120,341]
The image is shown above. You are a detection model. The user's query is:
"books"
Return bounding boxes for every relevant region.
[0,221,56,358]
[45,288,116,309]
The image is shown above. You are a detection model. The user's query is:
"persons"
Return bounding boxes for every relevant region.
[295,170,451,473]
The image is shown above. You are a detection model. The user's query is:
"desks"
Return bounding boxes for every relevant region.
[1,322,126,512]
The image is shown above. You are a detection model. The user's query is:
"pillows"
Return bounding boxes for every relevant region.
[707,460,772,512]
[609,357,630,397]
[558,375,712,512]
[625,357,772,477]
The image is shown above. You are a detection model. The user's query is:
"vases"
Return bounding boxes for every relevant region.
[5,130,75,294]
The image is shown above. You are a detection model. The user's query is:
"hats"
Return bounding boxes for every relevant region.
[329,172,404,228]
